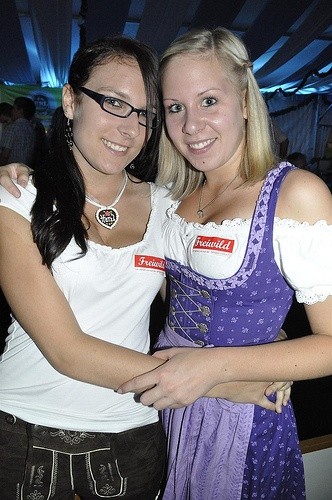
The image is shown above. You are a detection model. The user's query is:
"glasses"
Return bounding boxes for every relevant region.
[0,114,8,124]
[73,86,161,129]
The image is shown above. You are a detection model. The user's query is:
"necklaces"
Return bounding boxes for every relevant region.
[196,171,239,218]
[83,167,128,231]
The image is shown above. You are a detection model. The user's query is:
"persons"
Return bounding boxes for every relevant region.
[0,95,65,168]
[0,36,296,500]
[270,117,307,171]
[0,24,332,500]
